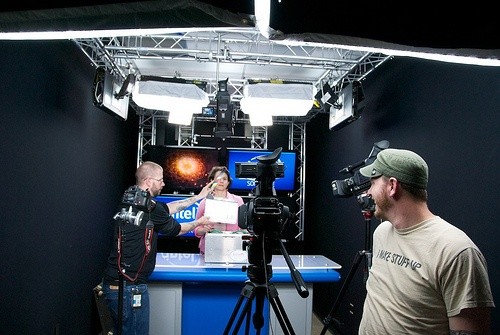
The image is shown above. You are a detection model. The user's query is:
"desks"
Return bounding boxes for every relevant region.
[146,253,343,335]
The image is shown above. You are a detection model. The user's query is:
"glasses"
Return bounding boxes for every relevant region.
[144,178,163,184]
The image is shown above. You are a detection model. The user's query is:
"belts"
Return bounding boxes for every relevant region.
[103,280,144,286]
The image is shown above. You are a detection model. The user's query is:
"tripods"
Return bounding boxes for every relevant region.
[317,208,374,335]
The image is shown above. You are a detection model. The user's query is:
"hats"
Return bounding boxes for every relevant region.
[359,149,428,188]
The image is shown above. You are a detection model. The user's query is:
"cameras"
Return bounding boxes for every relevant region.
[120,185,150,208]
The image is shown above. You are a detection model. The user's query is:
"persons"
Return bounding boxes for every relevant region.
[358,149,496,335]
[101,160,216,335]
[193,166,245,255]
